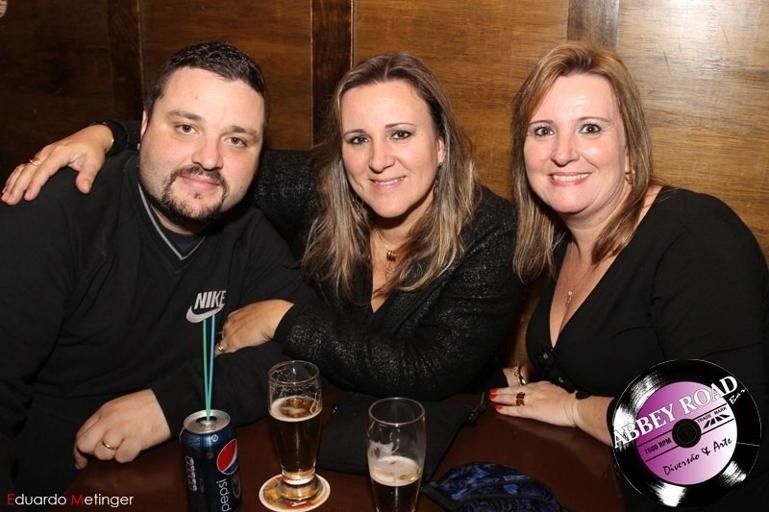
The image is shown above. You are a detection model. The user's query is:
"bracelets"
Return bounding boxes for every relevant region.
[511,366,526,386]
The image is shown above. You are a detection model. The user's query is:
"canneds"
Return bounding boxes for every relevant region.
[177,408,243,512]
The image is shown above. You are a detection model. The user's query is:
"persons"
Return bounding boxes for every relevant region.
[2,51,521,402]
[489,40,766,507]
[1,41,321,512]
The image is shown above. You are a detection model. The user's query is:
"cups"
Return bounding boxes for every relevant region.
[267,357,327,503]
[365,394,430,512]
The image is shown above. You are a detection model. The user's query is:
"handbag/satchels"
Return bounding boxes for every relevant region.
[317,391,485,485]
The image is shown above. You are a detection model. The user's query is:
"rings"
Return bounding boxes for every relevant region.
[101,442,121,450]
[514,392,527,405]
[216,341,227,353]
[27,156,40,165]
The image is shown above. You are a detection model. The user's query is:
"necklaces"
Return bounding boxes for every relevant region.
[373,225,403,262]
[567,238,595,302]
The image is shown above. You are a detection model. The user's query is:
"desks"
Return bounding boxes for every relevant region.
[55,391,629,512]
